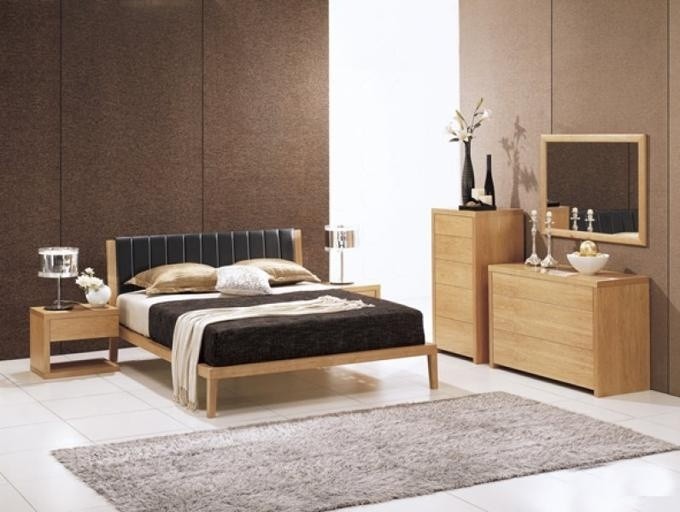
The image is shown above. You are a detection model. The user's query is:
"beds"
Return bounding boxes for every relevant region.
[571,209,639,238]
[104,227,441,421]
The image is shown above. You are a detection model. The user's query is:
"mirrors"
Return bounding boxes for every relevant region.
[539,133,651,249]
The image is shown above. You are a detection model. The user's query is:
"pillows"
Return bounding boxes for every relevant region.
[214,265,274,297]
[232,257,321,286]
[122,262,218,296]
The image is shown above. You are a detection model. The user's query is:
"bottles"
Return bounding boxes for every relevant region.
[484,154,495,208]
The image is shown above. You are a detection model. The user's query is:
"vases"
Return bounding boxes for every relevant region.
[483,153,497,210]
[458,141,477,205]
[84,285,112,309]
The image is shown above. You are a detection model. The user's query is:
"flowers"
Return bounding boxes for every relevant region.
[73,266,104,295]
[438,95,496,144]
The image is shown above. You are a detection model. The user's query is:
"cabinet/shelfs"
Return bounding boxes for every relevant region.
[547,206,569,230]
[429,205,527,366]
[484,260,653,400]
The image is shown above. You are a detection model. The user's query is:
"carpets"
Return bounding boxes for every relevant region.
[49,390,680,512]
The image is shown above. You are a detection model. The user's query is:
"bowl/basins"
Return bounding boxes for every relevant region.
[566,253,610,275]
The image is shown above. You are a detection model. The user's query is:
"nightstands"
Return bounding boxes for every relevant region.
[337,282,382,299]
[28,303,122,381]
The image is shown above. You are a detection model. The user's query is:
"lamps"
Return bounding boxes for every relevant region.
[30,246,82,311]
[320,222,359,287]
